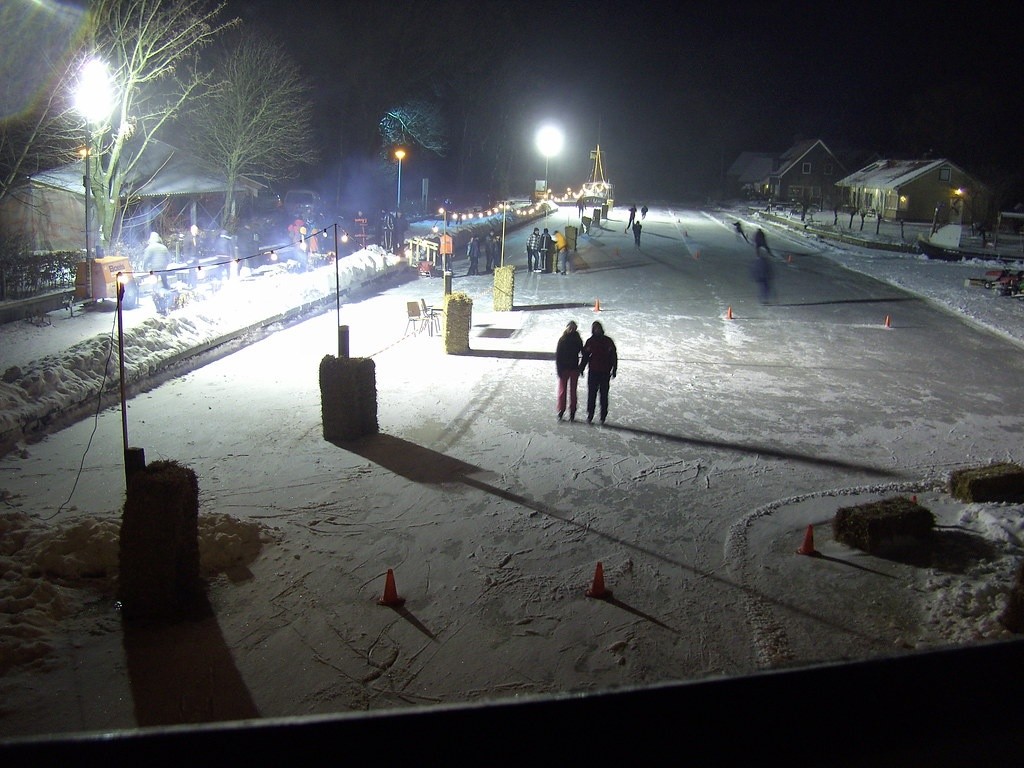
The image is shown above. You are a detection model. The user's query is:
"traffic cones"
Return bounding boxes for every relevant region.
[787,254,794,263]
[884,314,891,328]
[377,569,406,607]
[591,298,602,312]
[684,230,689,237]
[678,218,681,223]
[694,251,700,258]
[910,494,919,507]
[797,524,822,556]
[725,306,736,320]
[586,562,614,599]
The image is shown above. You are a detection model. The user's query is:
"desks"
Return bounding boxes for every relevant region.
[429,307,471,336]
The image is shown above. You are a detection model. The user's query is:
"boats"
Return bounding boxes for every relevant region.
[579,145,616,208]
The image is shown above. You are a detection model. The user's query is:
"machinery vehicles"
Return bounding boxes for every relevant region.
[529,177,550,205]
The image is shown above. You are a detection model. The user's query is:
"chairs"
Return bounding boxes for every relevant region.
[404,299,440,336]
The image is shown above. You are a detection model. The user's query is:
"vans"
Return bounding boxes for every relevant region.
[283,188,321,220]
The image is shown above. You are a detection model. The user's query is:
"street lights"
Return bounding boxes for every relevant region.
[76,61,104,299]
[536,121,561,198]
[394,148,406,210]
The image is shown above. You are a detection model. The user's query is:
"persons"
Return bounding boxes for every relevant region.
[633,221,642,251]
[467,236,482,276]
[526,228,567,275]
[455,206,464,225]
[641,205,648,220]
[576,196,586,218]
[216,229,233,280]
[755,228,776,260]
[143,232,171,290]
[732,221,749,243]
[625,204,637,234]
[486,229,501,274]
[556,320,617,425]
[182,224,200,288]
[357,207,409,257]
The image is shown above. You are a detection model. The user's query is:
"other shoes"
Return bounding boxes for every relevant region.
[533,269,542,273]
[557,410,563,422]
[569,410,575,422]
[561,271,566,275]
[586,412,594,423]
[600,415,607,424]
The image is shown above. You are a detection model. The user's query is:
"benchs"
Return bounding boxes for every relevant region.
[25,310,51,327]
